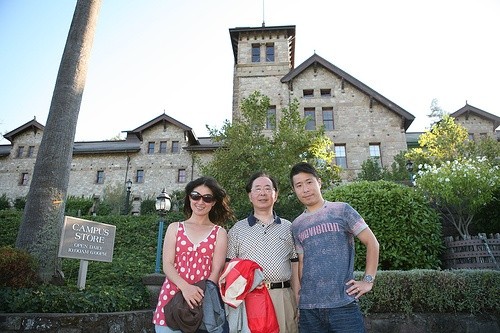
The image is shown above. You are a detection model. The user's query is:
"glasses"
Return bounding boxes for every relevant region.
[190,191,216,203]
[251,188,276,195]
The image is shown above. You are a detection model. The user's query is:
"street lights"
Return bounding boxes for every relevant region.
[155,188,172,273]
[123,179,133,215]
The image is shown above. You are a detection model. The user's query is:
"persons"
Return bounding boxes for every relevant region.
[153,177,234,333]
[289,163,380,333]
[225,171,300,333]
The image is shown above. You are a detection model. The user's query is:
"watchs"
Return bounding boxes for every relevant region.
[363,275,375,282]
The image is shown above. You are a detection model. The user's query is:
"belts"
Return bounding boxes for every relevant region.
[264,281,292,289]
